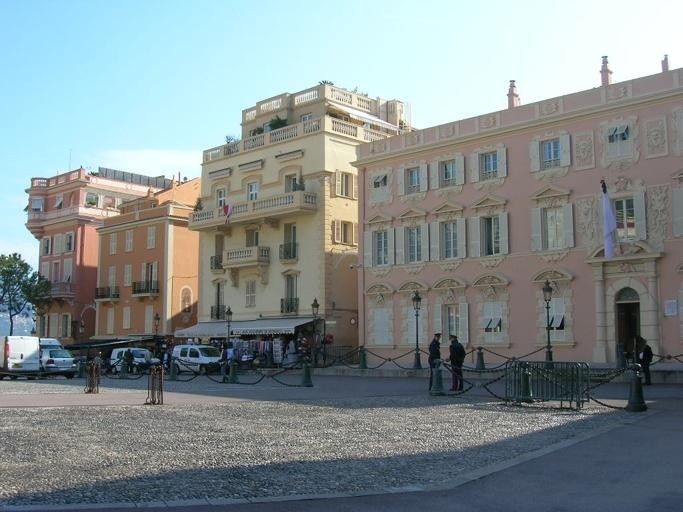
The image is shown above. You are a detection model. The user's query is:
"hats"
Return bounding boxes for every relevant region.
[448,334,458,340]
[434,333,442,337]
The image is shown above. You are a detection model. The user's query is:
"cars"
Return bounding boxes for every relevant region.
[38,350,78,379]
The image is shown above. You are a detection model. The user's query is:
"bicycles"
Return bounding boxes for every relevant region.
[239,349,268,365]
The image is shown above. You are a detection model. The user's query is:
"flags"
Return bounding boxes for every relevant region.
[600,183,618,263]
[222,202,234,225]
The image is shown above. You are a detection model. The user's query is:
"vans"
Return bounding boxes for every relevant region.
[38,338,63,350]
[171,345,221,374]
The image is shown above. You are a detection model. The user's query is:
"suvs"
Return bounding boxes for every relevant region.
[110,347,161,374]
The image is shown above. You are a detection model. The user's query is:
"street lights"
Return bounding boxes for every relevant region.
[79,323,85,378]
[154,313,161,359]
[225,306,233,367]
[311,297,319,367]
[412,289,421,369]
[542,280,553,369]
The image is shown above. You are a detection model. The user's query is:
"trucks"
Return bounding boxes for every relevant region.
[0,336,39,379]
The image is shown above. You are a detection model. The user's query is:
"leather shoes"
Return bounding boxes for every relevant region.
[450,388,464,391]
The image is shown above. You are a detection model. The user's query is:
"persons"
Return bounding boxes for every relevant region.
[216,342,229,383]
[445,332,464,390]
[427,331,442,386]
[125,348,132,372]
[143,348,152,361]
[636,339,654,384]
